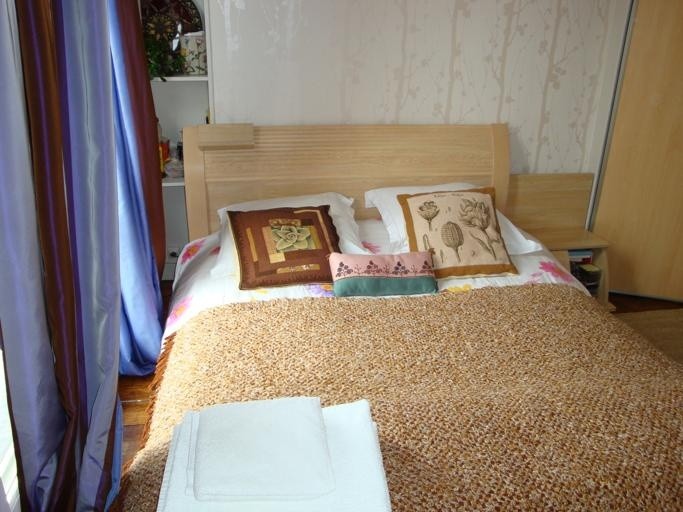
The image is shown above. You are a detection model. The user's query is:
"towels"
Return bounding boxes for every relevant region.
[155,397,392,512]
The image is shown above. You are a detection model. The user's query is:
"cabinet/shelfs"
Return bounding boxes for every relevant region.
[139,0,214,187]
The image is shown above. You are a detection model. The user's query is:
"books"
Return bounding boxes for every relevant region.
[179,30,207,76]
[551,249,594,276]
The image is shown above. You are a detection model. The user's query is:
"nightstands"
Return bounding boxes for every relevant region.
[524,228,616,314]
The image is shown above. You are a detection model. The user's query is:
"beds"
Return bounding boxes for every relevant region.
[123,124,682,512]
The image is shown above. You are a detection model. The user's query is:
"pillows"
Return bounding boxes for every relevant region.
[211,191,372,288]
[327,251,439,297]
[223,202,342,291]
[365,183,543,256]
[396,186,521,278]
